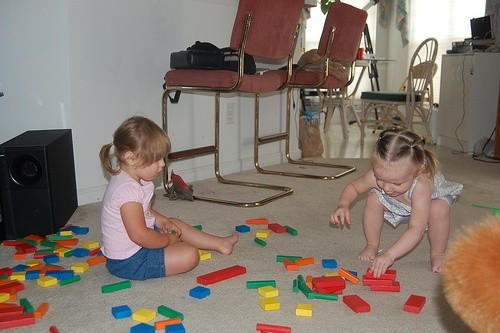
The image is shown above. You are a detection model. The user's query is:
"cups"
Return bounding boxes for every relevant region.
[357,47,364,59]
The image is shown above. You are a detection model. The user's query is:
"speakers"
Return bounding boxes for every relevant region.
[0,129,78,242]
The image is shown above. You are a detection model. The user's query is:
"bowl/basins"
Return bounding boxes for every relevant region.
[452,42,472,52]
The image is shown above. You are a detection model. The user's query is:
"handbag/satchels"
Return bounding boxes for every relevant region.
[162,40,257,103]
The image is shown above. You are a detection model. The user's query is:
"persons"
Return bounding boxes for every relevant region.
[329,129,463,279]
[100,117,238,279]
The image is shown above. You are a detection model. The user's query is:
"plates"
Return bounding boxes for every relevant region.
[363,54,375,59]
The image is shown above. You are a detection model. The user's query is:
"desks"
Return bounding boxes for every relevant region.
[325,58,395,140]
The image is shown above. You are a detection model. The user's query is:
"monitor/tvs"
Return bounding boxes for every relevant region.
[470,15,494,49]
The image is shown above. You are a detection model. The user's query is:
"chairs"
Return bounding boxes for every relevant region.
[254,2,368,180]
[361,38,439,147]
[162,0,305,207]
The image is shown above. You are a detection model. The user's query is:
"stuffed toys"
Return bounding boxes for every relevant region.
[439,215,500,333]
